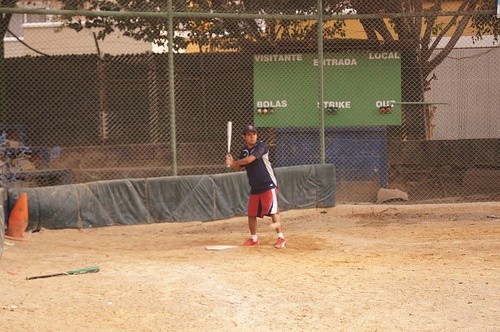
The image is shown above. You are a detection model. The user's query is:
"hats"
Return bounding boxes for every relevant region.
[242,125,257,135]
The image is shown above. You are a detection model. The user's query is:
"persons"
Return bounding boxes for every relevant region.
[225,124,286,250]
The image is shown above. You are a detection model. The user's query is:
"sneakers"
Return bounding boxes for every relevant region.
[243,238,259,246]
[275,237,286,248]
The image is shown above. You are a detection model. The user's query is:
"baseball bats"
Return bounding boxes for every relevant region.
[25,266,99,281]
[204,244,273,252]
[226,120,233,169]
